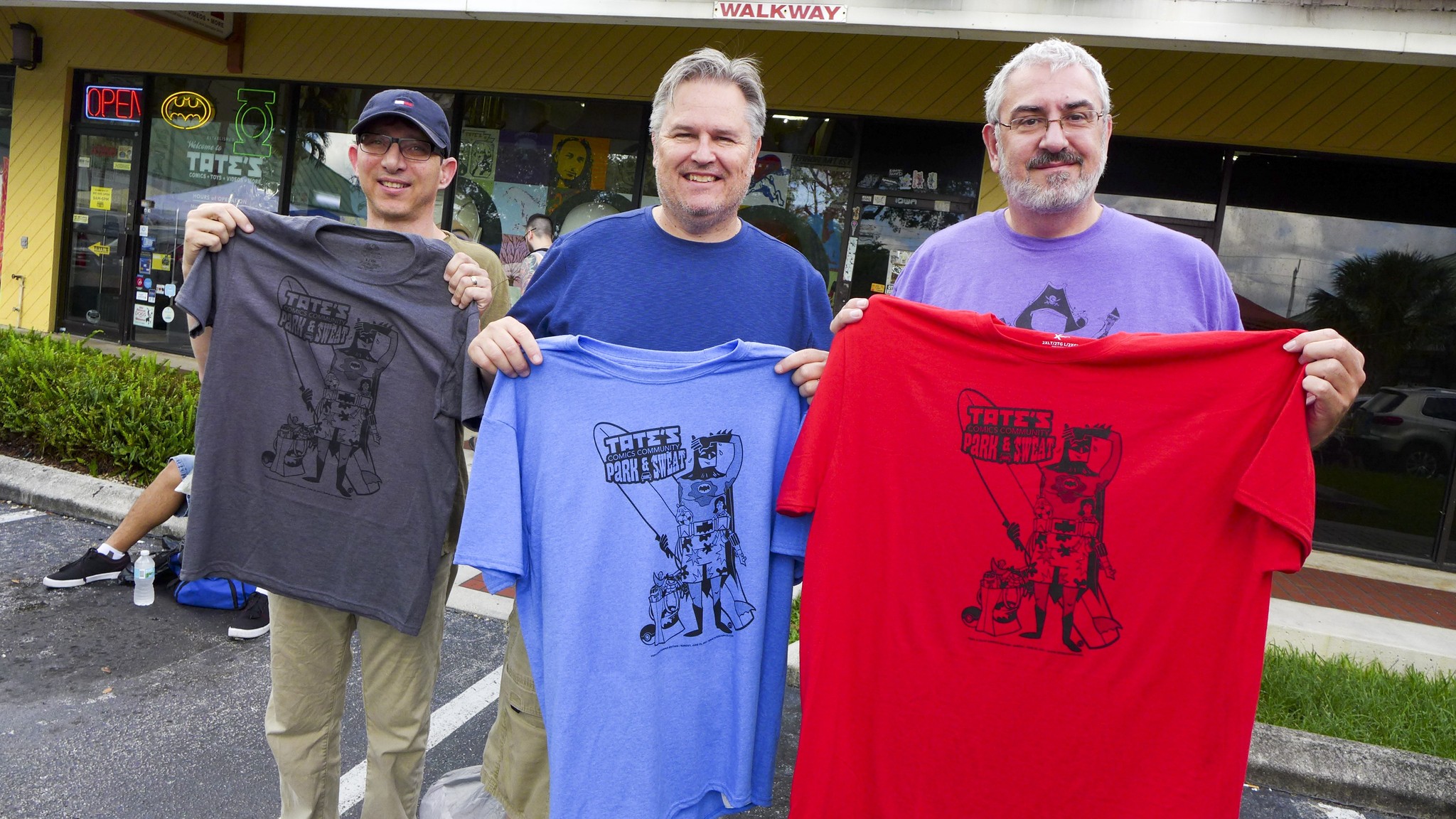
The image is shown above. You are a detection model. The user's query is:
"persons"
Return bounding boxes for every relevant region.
[815,41,1368,449]
[42,453,270,638]
[464,53,829,819]
[182,90,508,819]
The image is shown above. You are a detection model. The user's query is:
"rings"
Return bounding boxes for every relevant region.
[469,275,478,286]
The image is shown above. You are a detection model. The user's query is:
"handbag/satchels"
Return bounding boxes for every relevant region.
[116,534,179,587]
[168,552,256,611]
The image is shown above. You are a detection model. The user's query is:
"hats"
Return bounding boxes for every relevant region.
[350,89,452,158]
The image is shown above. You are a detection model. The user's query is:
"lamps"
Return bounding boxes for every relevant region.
[10,18,46,69]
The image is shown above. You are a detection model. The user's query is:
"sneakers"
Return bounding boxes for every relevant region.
[42,547,131,588]
[228,591,270,638]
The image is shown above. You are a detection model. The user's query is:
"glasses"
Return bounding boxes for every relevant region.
[358,130,448,162]
[996,111,1104,138]
[523,227,537,242]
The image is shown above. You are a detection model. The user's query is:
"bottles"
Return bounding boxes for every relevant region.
[133,550,155,606]
